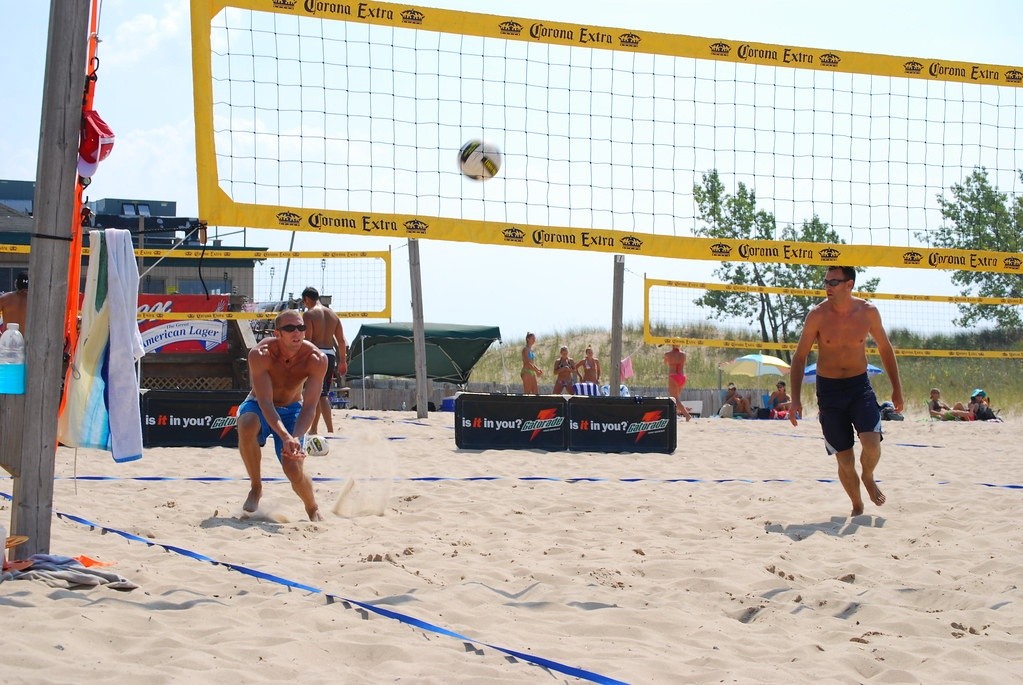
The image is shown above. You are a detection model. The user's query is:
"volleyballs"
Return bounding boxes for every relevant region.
[458,138,501,179]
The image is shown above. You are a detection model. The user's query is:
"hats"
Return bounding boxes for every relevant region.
[728,385,736,389]
[777,381,785,387]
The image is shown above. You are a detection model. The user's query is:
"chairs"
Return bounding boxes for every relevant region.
[601,384,631,397]
[983,397,1004,423]
[719,389,748,419]
[925,398,962,422]
[762,394,800,420]
[573,382,601,396]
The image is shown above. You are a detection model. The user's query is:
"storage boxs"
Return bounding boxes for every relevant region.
[441,396,455,412]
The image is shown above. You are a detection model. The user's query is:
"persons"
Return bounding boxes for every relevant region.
[664,338,692,421]
[928,388,1003,422]
[717,382,753,418]
[300,287,347,435]
[521,332,543,395]
[236,310,328,522]
[0,274,32,337]
[553,346,577,395]
[789,265,903,516]
[576,345,600,384]
[768,381,792,419]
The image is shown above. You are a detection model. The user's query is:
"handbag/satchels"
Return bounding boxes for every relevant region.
[769,409,789,419]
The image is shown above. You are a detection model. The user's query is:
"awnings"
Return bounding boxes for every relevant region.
[346,324,509,410]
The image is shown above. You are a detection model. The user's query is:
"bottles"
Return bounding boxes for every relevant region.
[0,323,24,364]
[402,402,406,411]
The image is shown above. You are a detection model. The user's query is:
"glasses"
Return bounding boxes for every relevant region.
[278,324,306,332]
[777,386,783,388]
[824,279,849,286]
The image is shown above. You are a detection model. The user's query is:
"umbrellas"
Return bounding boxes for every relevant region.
[803,361,884,382]
[718,350,791,408]
